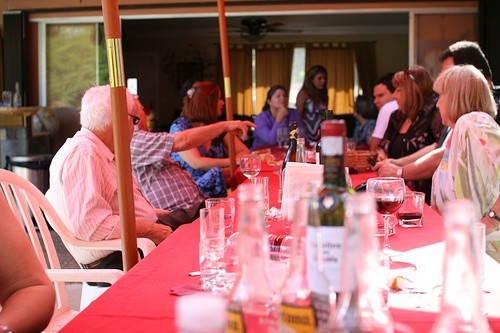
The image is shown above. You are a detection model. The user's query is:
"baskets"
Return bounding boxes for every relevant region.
[346,149,378,170]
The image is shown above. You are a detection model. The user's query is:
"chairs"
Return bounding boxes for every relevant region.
[0,168,157,333]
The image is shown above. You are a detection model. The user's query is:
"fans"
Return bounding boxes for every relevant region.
[211,17,303,43]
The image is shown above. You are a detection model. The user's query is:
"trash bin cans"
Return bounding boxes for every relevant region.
[7,154,53,228]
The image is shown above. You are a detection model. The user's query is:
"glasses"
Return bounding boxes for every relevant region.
[127,114,140,125]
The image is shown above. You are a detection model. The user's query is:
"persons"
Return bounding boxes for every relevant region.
[168,81,250,198]
[49,84,174,287]
[296,65,329,145]
[250,85,306,153]
[352,39,500,264]
[0,188,56,333]
[130,94,256,228]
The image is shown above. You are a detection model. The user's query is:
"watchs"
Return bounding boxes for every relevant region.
[487,208,500,223]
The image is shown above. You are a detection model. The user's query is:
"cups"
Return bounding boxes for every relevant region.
[372,209,396,237]
[397,190,425,229]
[480,222,486,289]
[198,238,239,292]
[346,138,357,152]
[2,91,12,108]
[248,176,270,216]
[199,207,226,265]
[205,197,237,239]
[173,293,228,333]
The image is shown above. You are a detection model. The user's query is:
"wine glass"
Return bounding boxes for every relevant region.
[365,175,406,250]
[239,153,262,185]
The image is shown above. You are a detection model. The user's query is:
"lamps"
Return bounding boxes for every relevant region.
[240,19,266,41]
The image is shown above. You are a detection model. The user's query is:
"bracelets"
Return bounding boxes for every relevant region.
[397,167,404,178]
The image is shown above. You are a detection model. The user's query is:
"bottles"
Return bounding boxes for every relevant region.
[226,230,296,253]
[227,116,494,333]
[12,81,22,108]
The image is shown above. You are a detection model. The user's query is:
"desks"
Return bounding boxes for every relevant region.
[55,142,500,333]
